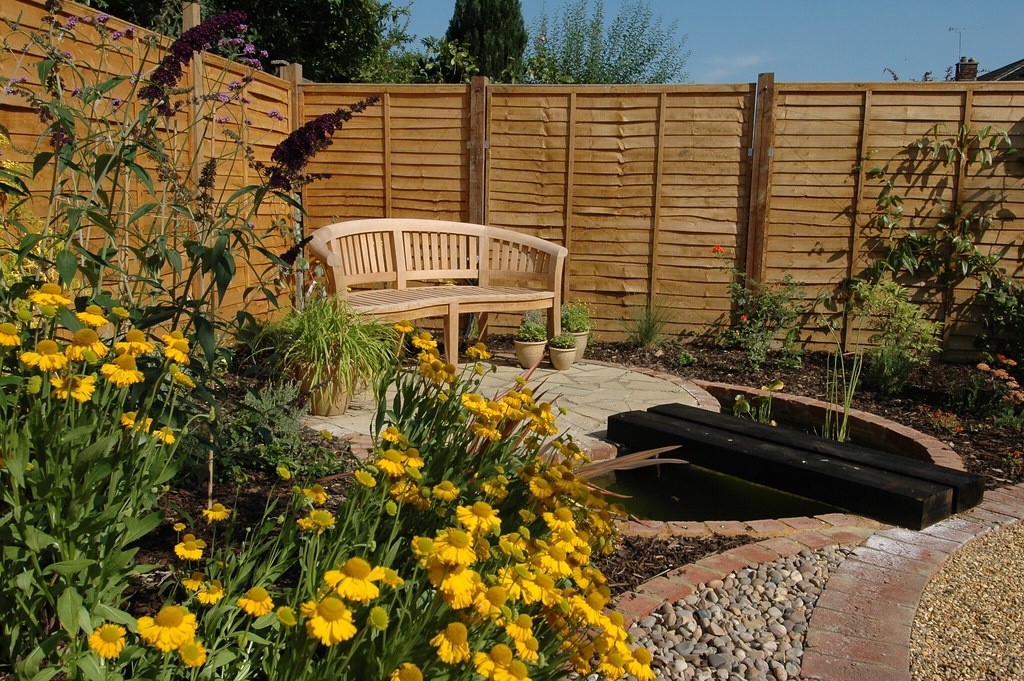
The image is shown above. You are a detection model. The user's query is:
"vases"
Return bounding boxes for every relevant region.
[564,330,590,362]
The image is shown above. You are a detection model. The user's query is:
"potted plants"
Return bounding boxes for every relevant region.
[260,293,400,417]
[513,310,548,368]
[548,328,578,370]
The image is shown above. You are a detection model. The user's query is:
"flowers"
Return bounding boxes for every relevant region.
[559,298,598,334]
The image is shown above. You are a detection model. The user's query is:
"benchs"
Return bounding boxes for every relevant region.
[306,219,568,366]
